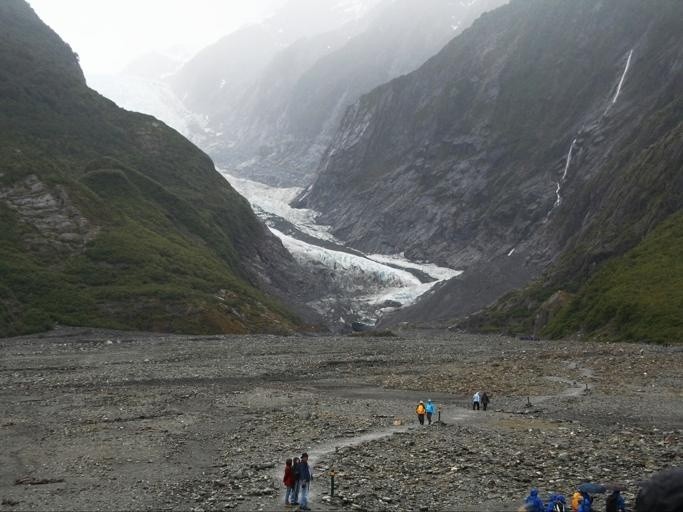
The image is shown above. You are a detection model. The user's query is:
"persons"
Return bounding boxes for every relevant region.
[481,392,490,410]
[425,398,435,425]
[524,488,626,512]
[283,459,292,504]
[636,472,683,512]
[291,458,300,505]
[415,400,426,425]
[299,453,314,511]
[473,392,480,410]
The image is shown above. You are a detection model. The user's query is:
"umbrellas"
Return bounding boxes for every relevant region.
[581,483,606,497]
[609,483,628,491]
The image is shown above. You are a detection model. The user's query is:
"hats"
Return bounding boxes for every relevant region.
[300,453,308,458]
